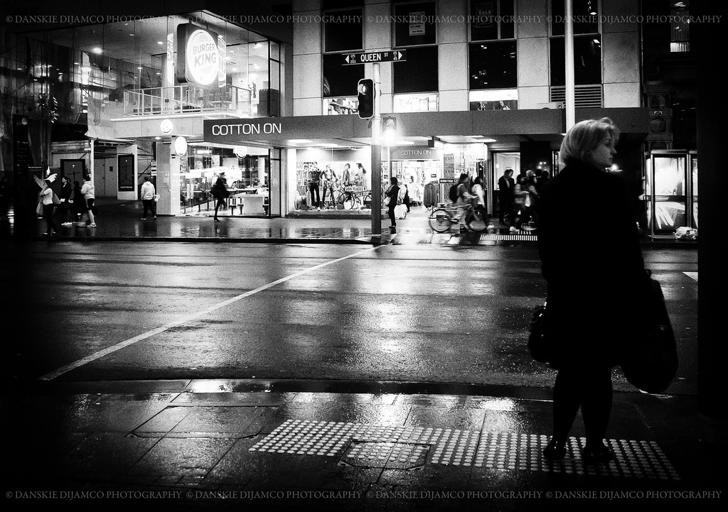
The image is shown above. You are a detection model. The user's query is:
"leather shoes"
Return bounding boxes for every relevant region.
[542,437,567,461]
[589,440,613,465]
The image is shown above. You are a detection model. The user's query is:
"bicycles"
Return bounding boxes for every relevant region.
[337,190,384,211]
[429,194,492,233]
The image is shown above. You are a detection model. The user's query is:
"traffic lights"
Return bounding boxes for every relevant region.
[357,79,373,118]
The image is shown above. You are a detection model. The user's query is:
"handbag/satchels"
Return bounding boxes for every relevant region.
[528,303,548,362]
[619,273,679,394]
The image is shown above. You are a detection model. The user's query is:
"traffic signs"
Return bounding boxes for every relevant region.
[339,48,407,66]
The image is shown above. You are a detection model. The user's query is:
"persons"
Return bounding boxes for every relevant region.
[498,168,549,233]
[40,179,59,236]
[384,177,400,228]
[341,163,353,208]
[139,176,157,221]
[352,163,366,208]
[450,174,478,230]
[532,117,645,465]
[465,176,494,229]
[309,167,321,211]
[79,174,97,228]
[319,165,339,210]
[58,176,72,222]
[216,172,226,184]
